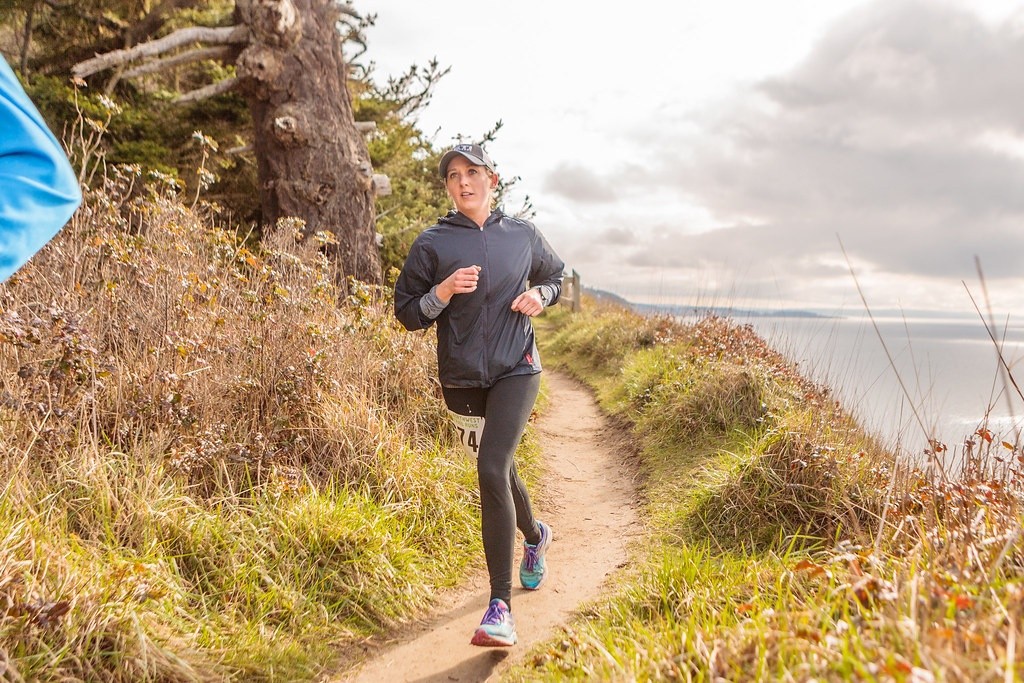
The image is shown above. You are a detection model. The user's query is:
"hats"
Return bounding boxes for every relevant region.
[438,144,498,176]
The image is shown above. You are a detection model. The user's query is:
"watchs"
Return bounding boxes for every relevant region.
[537,284,551,302]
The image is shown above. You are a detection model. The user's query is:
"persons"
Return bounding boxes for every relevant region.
[0,49,83,284]
[394,144,565,647]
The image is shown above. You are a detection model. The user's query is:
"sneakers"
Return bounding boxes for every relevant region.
[471,598,518,648]
[519,520,553,590]
